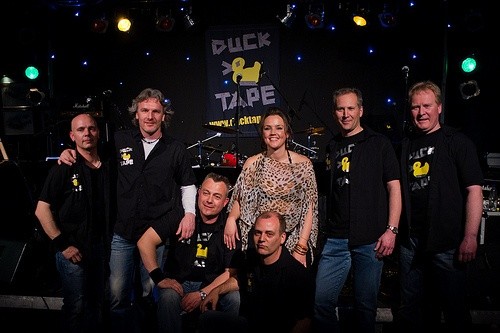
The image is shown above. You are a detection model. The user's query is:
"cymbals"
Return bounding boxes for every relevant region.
[203,125,235,134]
[294,127,325,135]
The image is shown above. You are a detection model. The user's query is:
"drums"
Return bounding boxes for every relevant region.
[221,151,241,167]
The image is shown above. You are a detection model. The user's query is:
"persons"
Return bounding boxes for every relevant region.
[314,88,401,333]
[35,111,117,333]
[57,88,198,333]
[137,171,241,333]
[398,82,486,333]
[200,211,316,333]
[223,107,319,274]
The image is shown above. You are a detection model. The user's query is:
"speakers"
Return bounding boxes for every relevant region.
[0,238,51,295]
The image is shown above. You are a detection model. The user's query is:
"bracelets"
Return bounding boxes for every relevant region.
[293,243,309,257]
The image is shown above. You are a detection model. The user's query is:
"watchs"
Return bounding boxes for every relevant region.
[199,289,208,301]
[386,225,399,235]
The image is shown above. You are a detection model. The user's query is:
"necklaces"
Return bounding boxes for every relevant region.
[86,159,101,170]
[141,137,161,144]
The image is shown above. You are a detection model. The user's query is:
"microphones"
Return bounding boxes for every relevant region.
[402,66,409,78]
[103,90,112,94]
[236,74,242,82]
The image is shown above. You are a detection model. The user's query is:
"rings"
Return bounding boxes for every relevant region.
[191,230,194,234]
